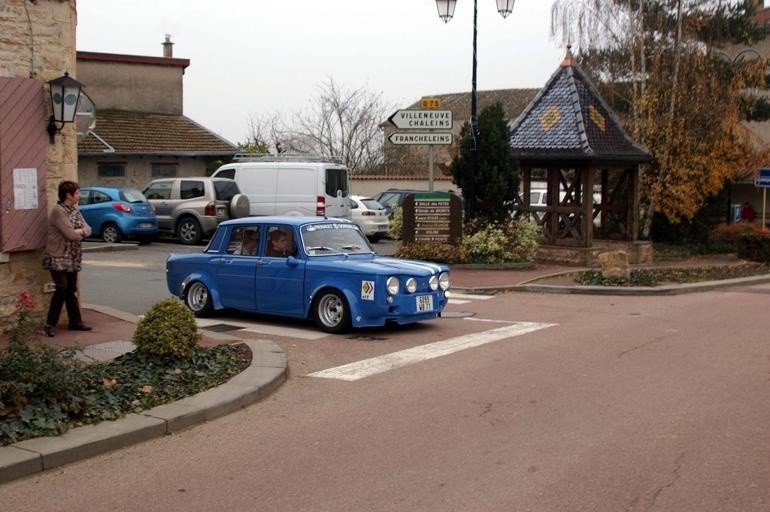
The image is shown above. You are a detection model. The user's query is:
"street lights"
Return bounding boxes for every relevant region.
[435,0,518,121]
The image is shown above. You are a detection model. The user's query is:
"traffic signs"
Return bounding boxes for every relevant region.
[389,110,454,131]
[387,135,452,145]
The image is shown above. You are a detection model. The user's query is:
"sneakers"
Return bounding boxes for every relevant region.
[68,323,93,331]
[43,324,56,337]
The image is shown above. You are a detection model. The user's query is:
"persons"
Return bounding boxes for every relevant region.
[39,181,93,337]
[233,230,259,256]
[267,231,291,257]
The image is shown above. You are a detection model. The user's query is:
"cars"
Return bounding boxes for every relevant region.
[168,217,456,334]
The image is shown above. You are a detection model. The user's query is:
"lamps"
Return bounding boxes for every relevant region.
[45,71,86,145]
